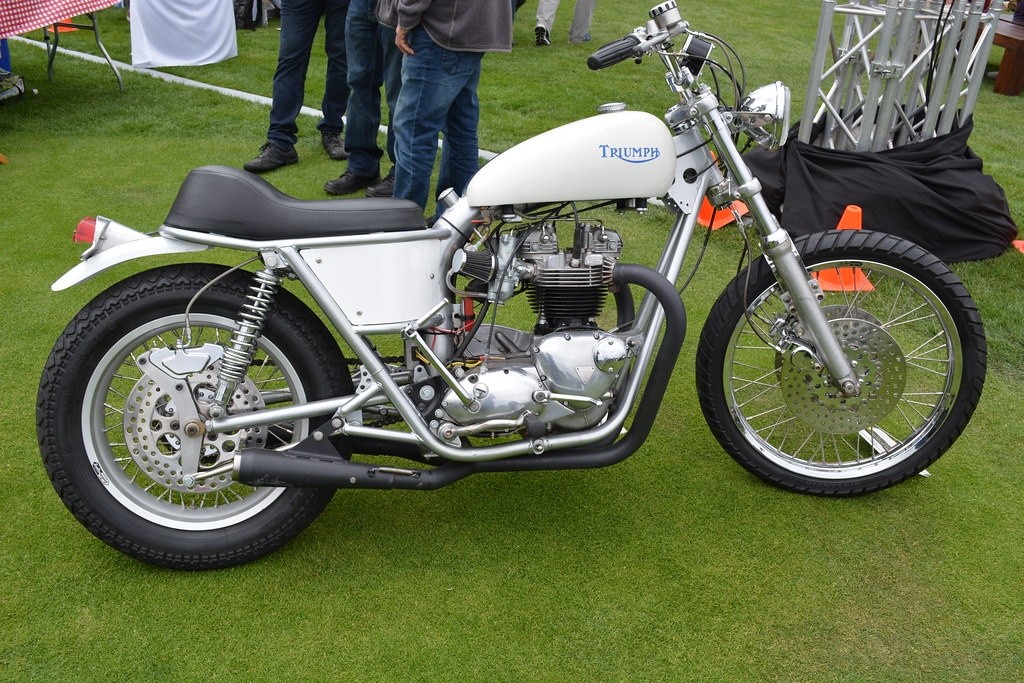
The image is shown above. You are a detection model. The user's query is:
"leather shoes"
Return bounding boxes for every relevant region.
[243,140,298,172]
[323,170,381,195]
[364,174,395,197]
[321,131,348,160]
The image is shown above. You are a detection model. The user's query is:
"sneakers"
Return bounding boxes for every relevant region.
[535,24,551,45]
[568,33,591,43]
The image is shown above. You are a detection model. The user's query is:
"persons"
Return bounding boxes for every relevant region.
[243,1,346,172]
[374,0,513,227]
[535,0,595,47]
[322,0,406,196]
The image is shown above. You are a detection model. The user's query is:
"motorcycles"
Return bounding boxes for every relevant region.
[33,0,988,572]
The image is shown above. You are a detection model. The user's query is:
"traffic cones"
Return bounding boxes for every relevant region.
[810,203,875,294]
[47,17,81,34]
[1012,238,1024,255]
[693,150,750,229]
